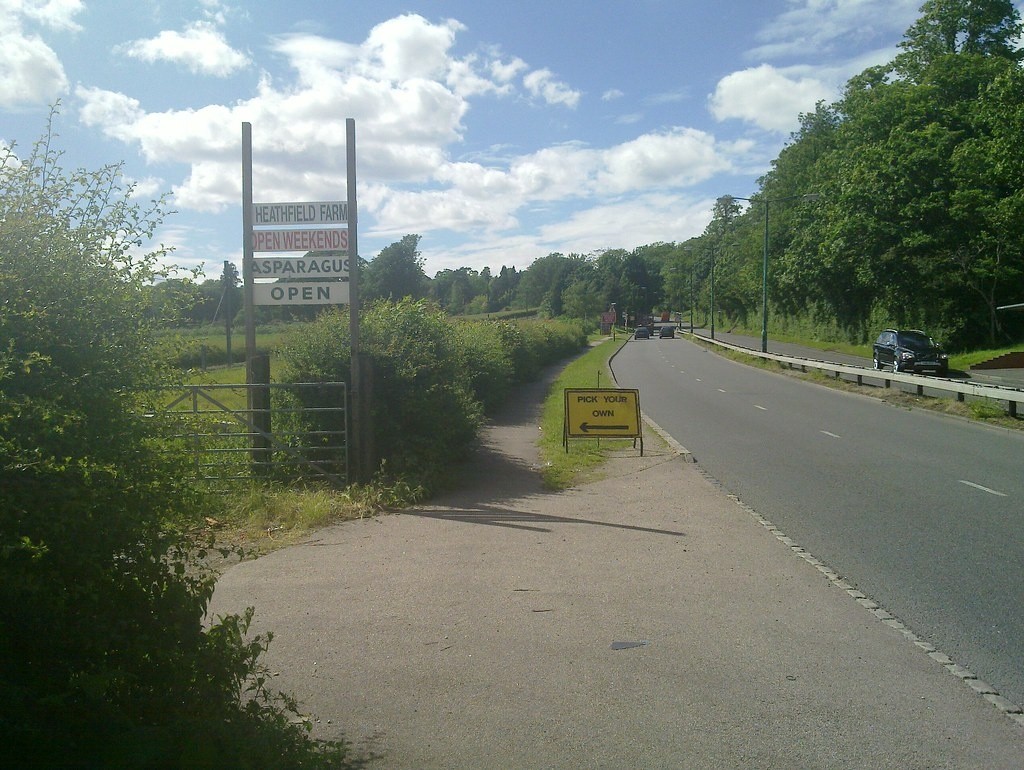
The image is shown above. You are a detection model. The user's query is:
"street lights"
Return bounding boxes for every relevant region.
[684,243,742,340]
[670,268,692,333]
[716,194,820,353]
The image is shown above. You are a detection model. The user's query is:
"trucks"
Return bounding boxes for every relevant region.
[639,318,654,336]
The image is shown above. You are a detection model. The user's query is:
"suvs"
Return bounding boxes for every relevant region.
[872,328,949,377]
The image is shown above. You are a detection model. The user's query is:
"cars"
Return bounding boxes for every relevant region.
[634,327,649,340]
[659,326,674,338]
